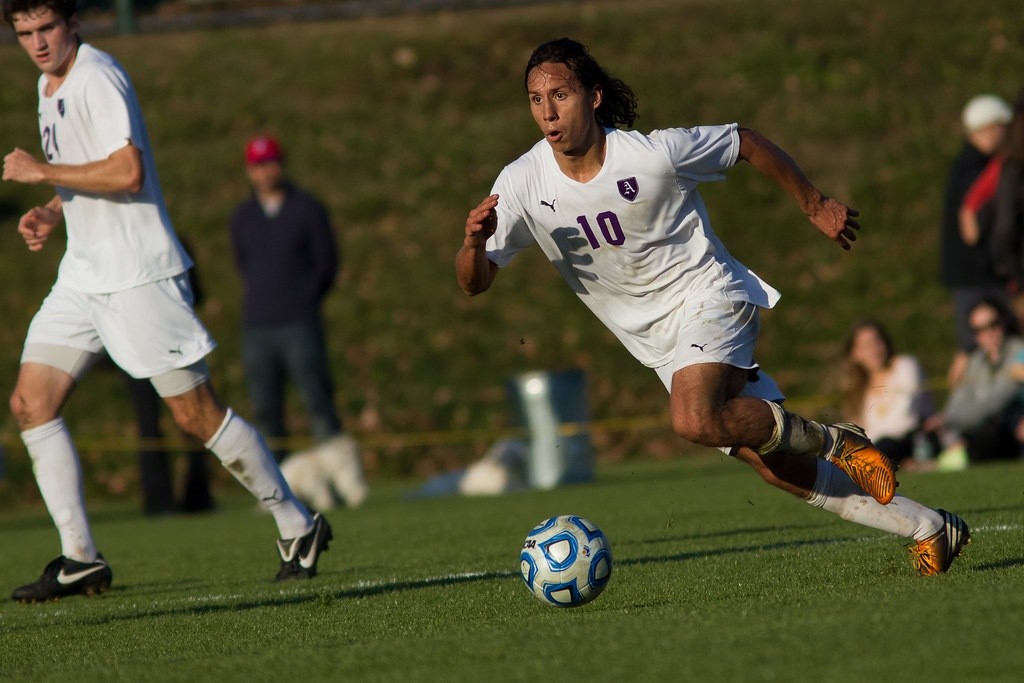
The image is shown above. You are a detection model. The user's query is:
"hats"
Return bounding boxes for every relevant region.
[962,93,1014,133]
[246,138,281,165]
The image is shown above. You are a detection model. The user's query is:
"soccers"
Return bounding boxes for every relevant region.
[520,514,613,609]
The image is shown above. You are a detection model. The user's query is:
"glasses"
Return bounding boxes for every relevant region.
[971,319,1001,335]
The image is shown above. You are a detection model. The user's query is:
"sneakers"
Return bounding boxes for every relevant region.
[823,424,898,505]
[12,552,111,603]
[274,513,333,579]
[909,508,971,577]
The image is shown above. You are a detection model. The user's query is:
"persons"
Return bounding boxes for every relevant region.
[229,133,339,459]
[929,95,1023,469]
[844,319,940,468]
[456,38,970,578]
[120,234,217,516]
[0,0,334,596]
